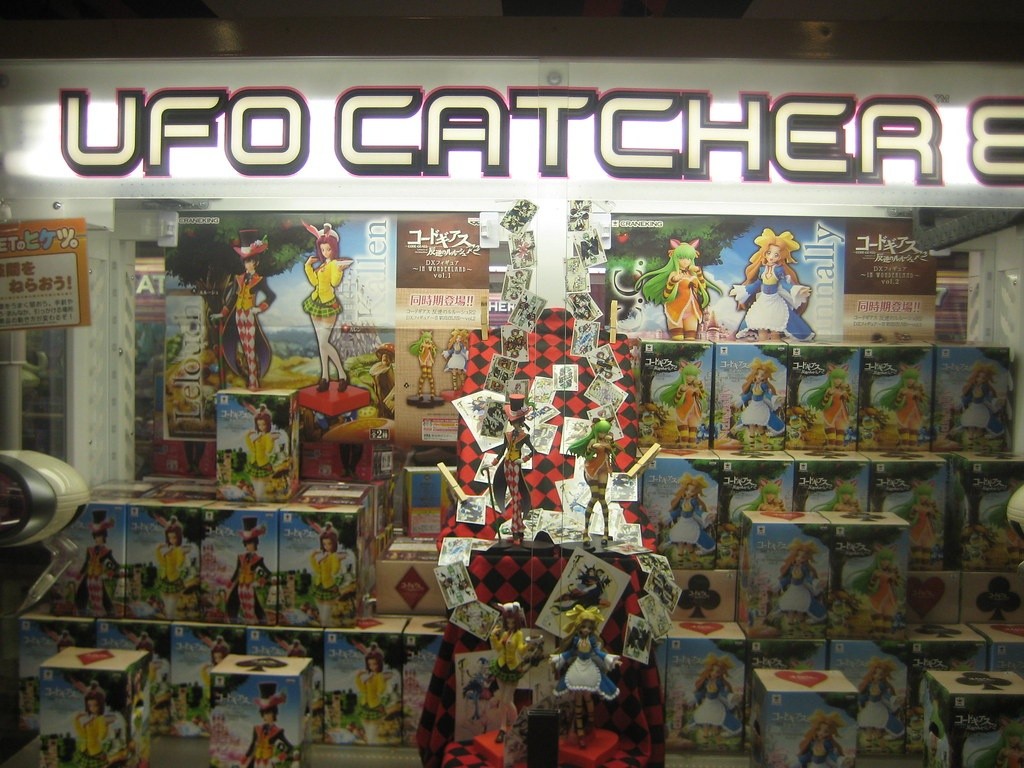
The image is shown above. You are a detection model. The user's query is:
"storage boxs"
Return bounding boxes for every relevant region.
[17,340,1024,768]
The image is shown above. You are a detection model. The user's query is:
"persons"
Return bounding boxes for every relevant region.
[483,394,536,546]
[567,416,617,548]
[547,606,623,747]
[489,601,545,744]
